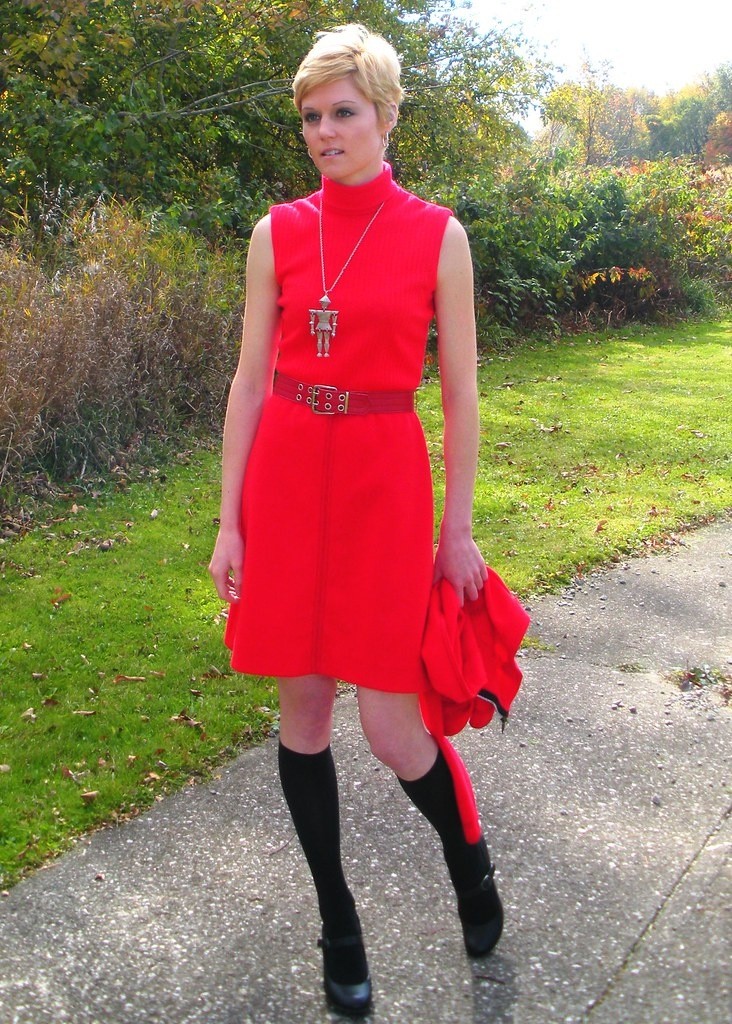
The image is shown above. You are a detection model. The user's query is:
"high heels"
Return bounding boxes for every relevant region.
[318,911,372,1017]
[443,835,504,957]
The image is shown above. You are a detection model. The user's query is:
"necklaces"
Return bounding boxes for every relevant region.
[309,187,385,358]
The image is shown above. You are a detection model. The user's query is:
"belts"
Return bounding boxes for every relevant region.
[273,374,420,417]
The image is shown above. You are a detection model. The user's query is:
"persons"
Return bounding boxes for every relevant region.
[208,21,530,1017]
